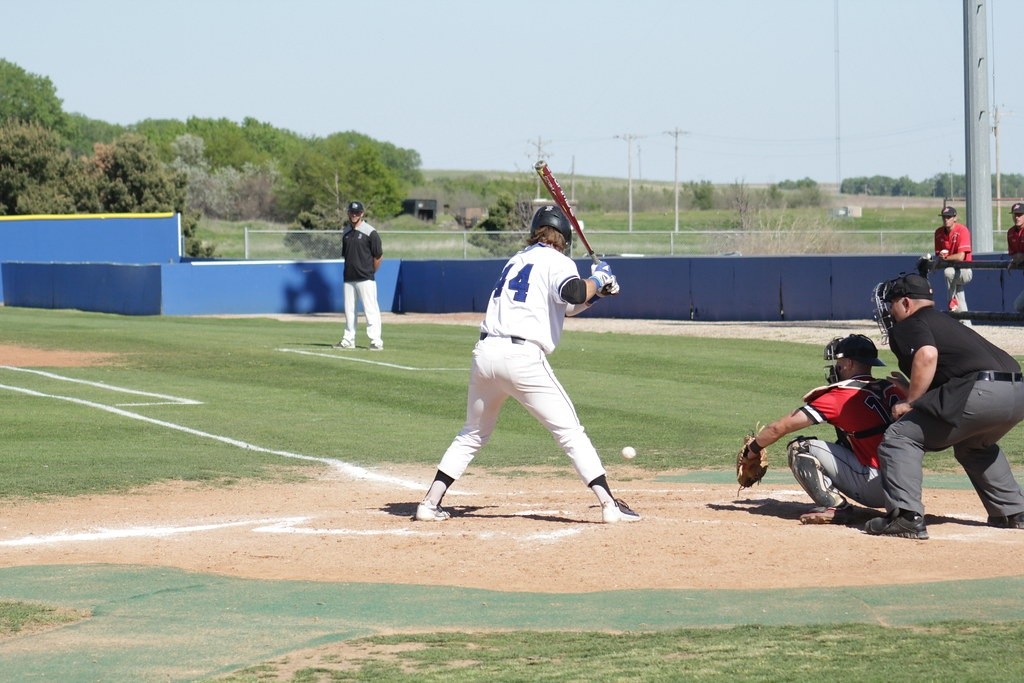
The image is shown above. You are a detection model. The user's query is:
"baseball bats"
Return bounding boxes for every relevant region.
[534,160,619,297]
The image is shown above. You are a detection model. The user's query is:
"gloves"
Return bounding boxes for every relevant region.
[589,260,612,290]
[595,274,620,297]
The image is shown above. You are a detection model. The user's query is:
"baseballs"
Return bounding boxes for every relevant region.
[622,446,636,459]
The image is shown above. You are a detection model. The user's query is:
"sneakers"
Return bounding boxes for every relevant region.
[602,500,642,523]
[865,509,928,540]
[800,505,856,524]
[416,503,451,521]
[948,297,959,312]
[987,513,1024,530]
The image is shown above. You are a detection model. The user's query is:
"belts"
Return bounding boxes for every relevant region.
[480,333,525,344]
[977,372,1024,382]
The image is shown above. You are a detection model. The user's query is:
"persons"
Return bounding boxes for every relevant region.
[332,201,384,352]
[934,206,973,326]
[1007,203,1024,311]
[415,204,641,522]
[741,334,906,524]
[865,272,1024,539]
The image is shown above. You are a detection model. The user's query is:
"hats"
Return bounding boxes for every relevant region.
[886,276,933,299]
[938,207,956,217]
[1009,203,1024,214]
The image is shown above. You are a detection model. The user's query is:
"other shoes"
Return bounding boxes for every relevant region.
[370,344,381,350]
[332,343,355,348]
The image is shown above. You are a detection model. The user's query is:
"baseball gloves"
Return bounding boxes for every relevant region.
[736,435,768,488]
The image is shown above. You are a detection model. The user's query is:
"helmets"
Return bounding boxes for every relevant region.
[346,202,364,212]
[530,206,572,244]
[838,335,887,366]
